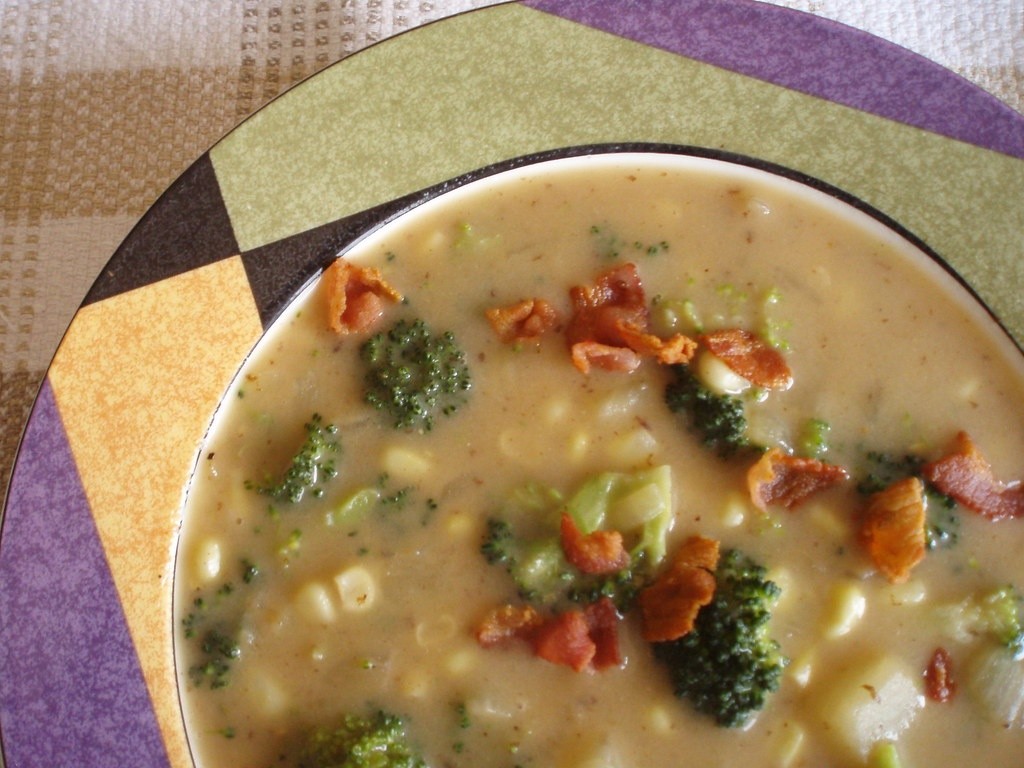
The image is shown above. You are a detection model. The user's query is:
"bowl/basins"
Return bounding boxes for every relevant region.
[3,3,1024,768]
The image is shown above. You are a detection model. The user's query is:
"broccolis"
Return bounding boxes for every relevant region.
[176,220,1024,768]
